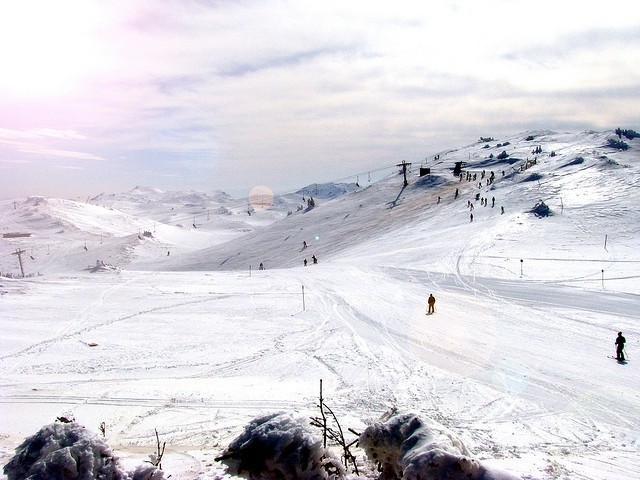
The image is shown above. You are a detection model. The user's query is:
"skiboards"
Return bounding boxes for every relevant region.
[607,355,628,364]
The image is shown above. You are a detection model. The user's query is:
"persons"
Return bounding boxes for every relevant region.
[428,293,435,313]
[302,240,318,265]
[612,332,626,359]
[436,168,504,219]
